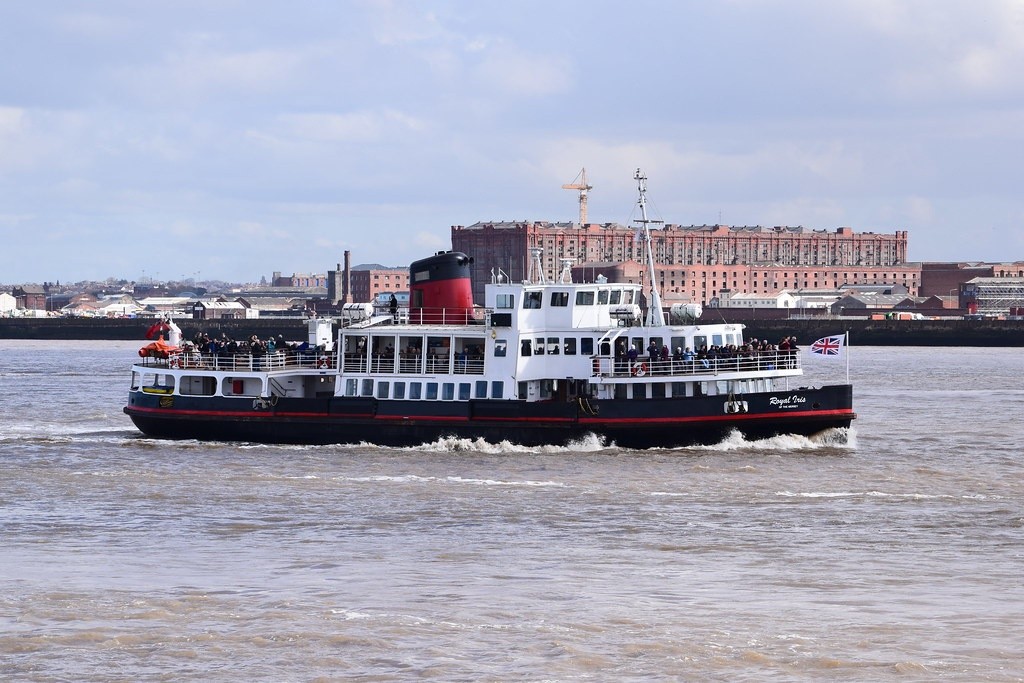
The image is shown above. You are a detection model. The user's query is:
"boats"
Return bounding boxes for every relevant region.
[124,166,858,447]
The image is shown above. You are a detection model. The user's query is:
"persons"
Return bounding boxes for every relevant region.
[390,294,398,324]
[647,341,659,375]
[526,295,539,309]
[626,344,638,375]
[356,342,484,375]
[615,341,626,376]
[673,336,797,374]
[178,331,338,371]
[660,345,668,375]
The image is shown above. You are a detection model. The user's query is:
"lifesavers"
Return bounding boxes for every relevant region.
[632,362,647,377]
[319,355,331,369]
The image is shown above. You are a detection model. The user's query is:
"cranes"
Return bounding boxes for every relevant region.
[563,166,596,228]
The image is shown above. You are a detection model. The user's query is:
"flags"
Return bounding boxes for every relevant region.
[809,333,845,360]
[631,229,640,248]
